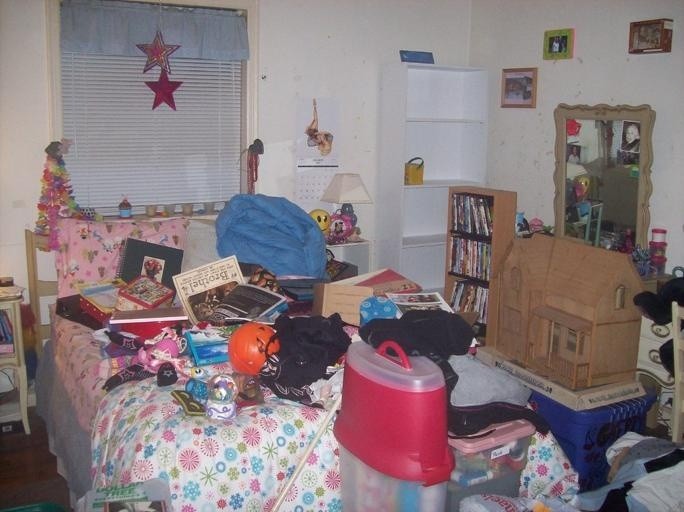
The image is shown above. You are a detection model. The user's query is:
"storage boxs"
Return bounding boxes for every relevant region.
[529,389,659,494]
[444,417,536,511]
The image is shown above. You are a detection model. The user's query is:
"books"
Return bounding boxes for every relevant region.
[116,236,184,304]
[109,307,188,325]
[448,194,493,324]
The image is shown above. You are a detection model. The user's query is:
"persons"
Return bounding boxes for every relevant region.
[622,124,640,152]
[567,147,579,163]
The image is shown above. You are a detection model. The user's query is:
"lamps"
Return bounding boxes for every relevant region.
[318,173,374,233]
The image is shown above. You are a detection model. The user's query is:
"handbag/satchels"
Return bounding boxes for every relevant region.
[413,350,549,434]
[262,313,352,388]
[405,157,424,185]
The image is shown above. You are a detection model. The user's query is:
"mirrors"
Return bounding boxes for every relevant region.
[551,102,654,256]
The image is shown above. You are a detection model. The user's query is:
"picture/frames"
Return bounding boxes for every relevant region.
[629,18,672,54]
[542,28,574,59]
[499,68,536,108]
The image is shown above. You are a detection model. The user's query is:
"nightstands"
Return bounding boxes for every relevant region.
[0,293,33,437]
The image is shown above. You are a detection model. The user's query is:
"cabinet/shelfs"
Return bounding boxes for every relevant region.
[441,184,518,348]
[373,63,489,292]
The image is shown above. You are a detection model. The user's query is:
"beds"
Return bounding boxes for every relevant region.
[24,212,532,510]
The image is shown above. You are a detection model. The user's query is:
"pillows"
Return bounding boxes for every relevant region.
[178,218,221,279]
[48,216,191,300]
